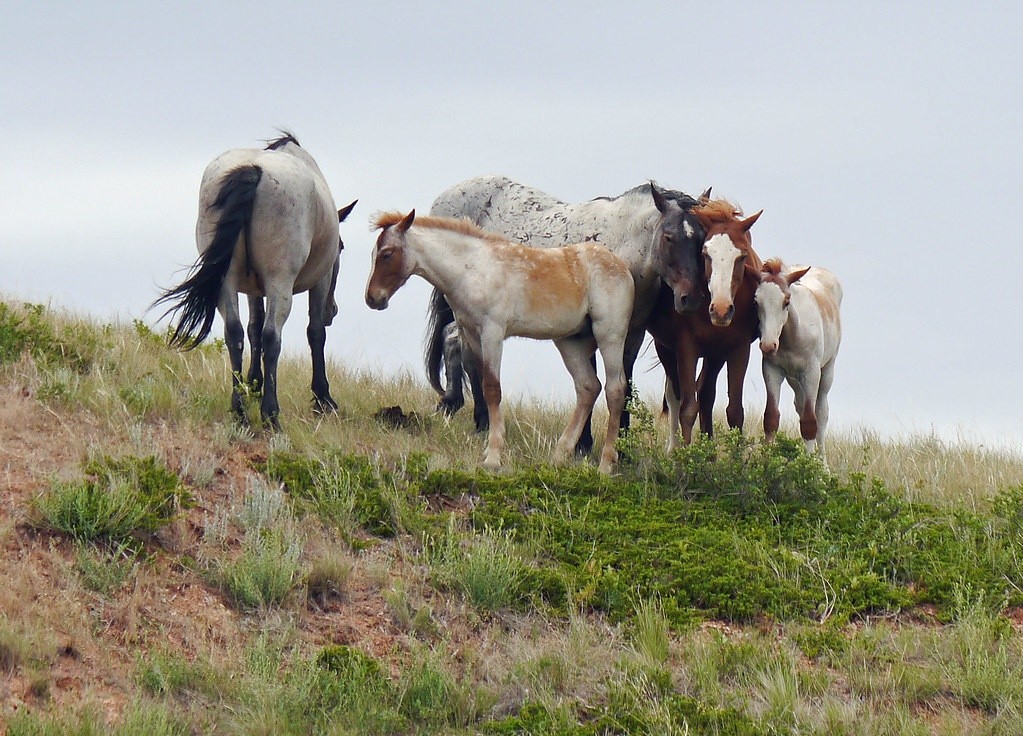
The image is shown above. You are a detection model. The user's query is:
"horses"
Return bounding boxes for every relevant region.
[364,208,635,477]
[644,197,764,465]
[744,256,843,483]
[144,127,359,436]
[419,178,714,465]
